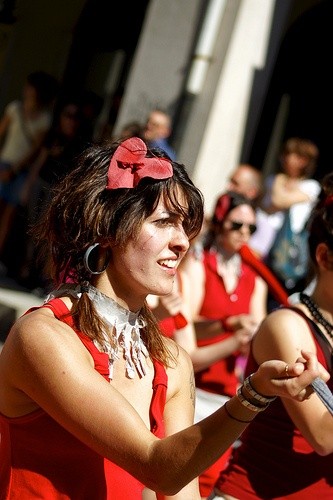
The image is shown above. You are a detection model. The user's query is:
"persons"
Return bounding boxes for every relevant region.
[174,190,267,398]
[306,174,333,283]
[226,164,260,200]
[247,138,321,267]
[144,251,205,342]
[207,198,333,500]
[0,70,104,282]
[0,134,330,500]
[142,111,175,160]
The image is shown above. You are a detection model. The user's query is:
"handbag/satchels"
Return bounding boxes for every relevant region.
[271,209,310,279]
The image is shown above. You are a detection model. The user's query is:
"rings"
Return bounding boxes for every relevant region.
[286,365,289,376]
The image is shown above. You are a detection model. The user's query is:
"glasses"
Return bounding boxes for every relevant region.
[224,219,256,235]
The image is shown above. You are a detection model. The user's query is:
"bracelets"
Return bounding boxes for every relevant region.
[243,373,276,403]
[236,384,269,412]
[224,402,252,423]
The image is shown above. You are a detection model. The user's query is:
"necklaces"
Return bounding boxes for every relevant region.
[44,281,150,379]
[299,291,333,338]
